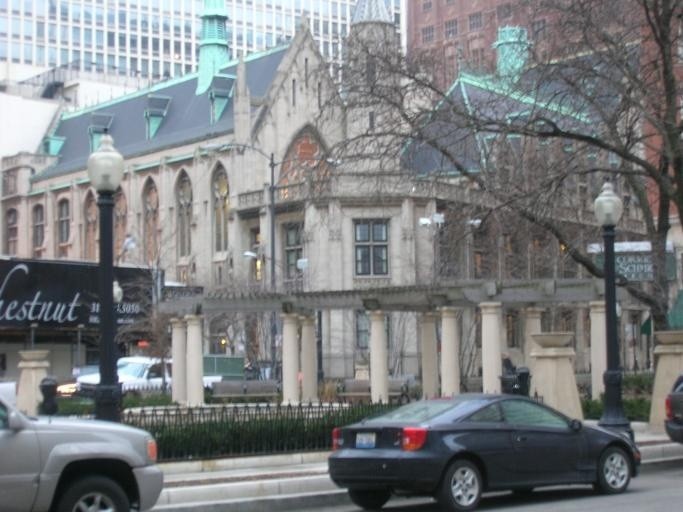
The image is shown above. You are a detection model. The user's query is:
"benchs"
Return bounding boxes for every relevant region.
[336,378,410,407]
[208,379,279,406]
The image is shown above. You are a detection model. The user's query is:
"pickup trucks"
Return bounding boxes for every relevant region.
[76,355,222,397]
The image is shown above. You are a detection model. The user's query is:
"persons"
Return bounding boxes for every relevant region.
[244,357,253,380]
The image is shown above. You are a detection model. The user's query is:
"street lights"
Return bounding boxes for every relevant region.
[113,278,124,318]
[87,128,125,423]
[592,177,635,444]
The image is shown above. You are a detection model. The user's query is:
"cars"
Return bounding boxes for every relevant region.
[326,392,640,509]
[663,375,682,444]
[0,401,165,512]
